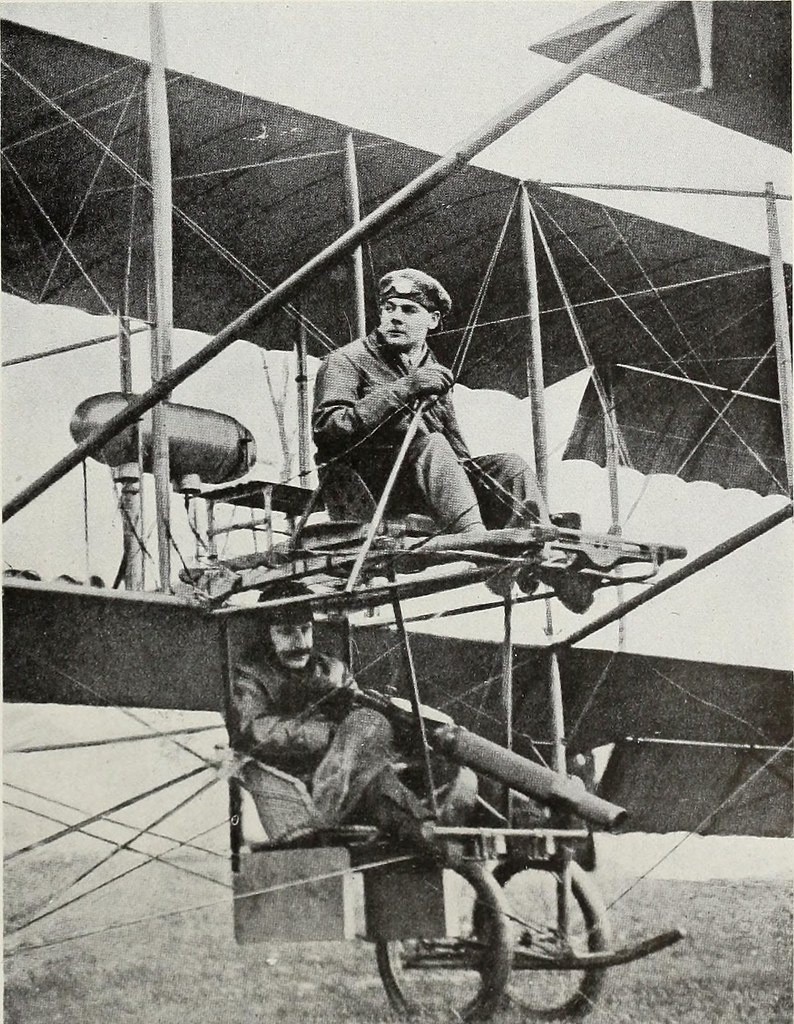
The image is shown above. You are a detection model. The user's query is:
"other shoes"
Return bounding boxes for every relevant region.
[555,525,622,614]
[480,499,540,595]
[433,766,479,867]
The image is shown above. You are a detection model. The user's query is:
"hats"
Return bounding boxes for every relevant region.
[378,267,452,317]
[254,579,317,626]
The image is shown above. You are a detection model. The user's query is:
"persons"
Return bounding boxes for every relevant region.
[312,268,622,616]
[228,578,496,863]
